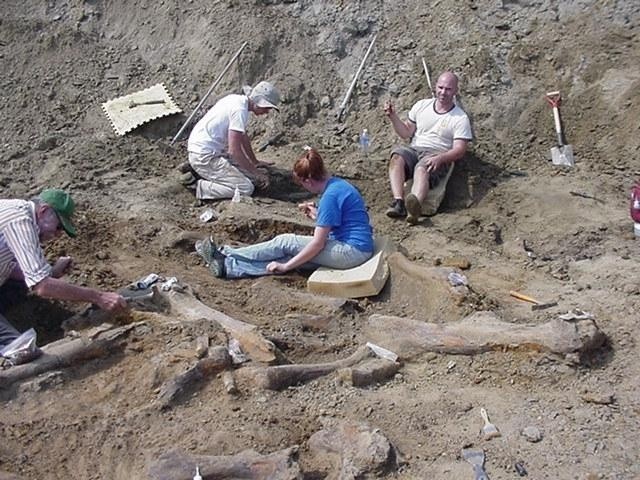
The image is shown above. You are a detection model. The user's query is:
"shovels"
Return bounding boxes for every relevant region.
[545,90,574,168]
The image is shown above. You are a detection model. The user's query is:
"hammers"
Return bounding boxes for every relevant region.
[129,99,164,108]
[509,290,557,311]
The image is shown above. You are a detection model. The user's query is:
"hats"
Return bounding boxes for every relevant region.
[243,81,282,114]
[39,189,76,238]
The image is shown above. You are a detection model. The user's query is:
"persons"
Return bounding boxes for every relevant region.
[194,148,373,279]
[383,72,473,224]
[0,188,128,358]
[177,80,281,200]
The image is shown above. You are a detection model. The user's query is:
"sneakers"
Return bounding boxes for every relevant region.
[386,198,406,217]
[406,194,420,223]
[195,237,226,276]
[175,162,198,190]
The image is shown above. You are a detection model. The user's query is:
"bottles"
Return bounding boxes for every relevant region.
[232,183,241,203]
[360,128,371,158]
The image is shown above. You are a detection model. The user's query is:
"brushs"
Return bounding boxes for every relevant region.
[481,408,501,437]
[461,448,489,480]
